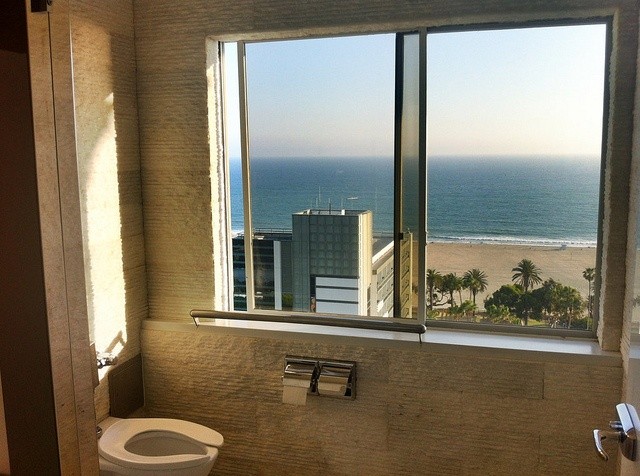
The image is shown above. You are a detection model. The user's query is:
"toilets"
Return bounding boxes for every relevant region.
[96,416,224,476]
